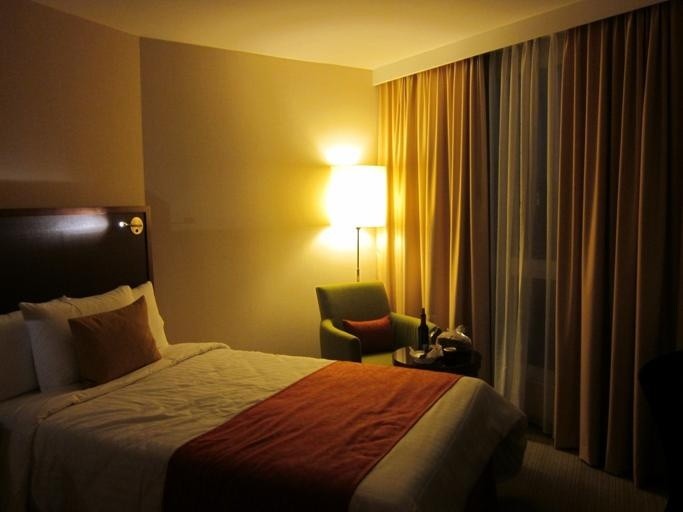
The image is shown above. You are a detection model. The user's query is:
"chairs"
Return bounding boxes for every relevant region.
[315,283,436,367]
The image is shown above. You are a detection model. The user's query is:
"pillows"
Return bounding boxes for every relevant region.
[0,281,170,402]
[345,315,395,350]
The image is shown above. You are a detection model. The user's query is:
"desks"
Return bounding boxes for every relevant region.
[392,343,482,378]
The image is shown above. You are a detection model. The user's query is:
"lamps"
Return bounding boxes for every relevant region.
[330,164,386,281]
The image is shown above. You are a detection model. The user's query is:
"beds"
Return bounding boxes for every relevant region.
[0,206,526,512]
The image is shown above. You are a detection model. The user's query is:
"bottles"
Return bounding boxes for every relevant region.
[417,308,429,354]
